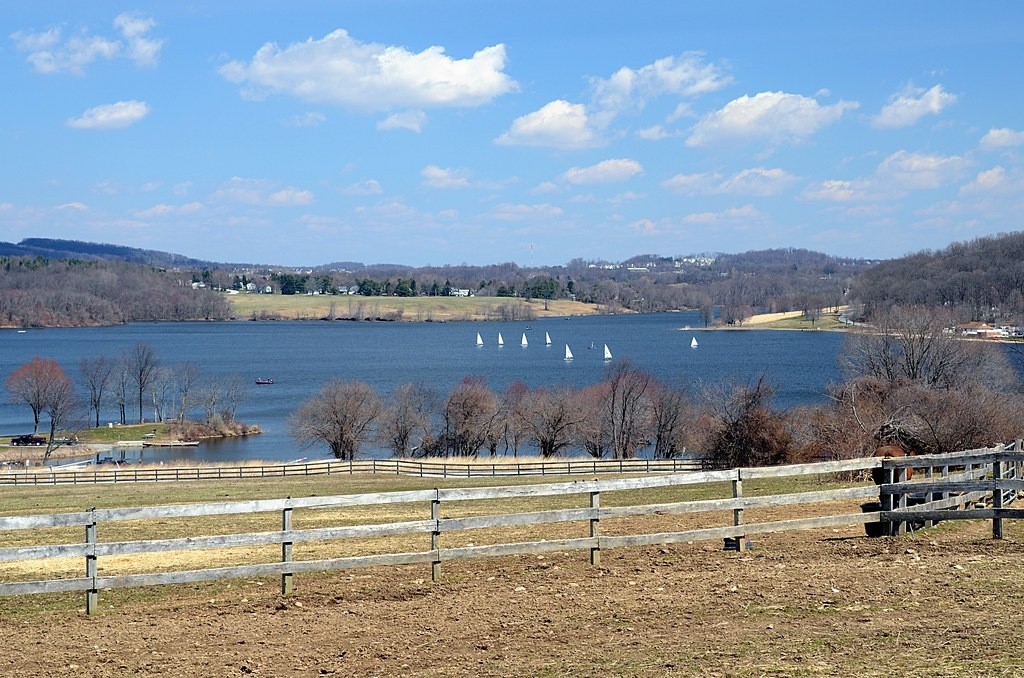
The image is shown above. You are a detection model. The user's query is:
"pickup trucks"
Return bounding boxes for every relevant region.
[11,434,46,446]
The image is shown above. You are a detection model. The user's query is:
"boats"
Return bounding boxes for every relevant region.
[18,331,26,333]
[256,381,273,384]
[565,317,571,320]
[143,441,200,446]
[588,346,598,350]
[526,326,532,330]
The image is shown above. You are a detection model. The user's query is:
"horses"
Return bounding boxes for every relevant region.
[872,445,916,506]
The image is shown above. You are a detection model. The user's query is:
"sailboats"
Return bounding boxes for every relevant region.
[498,333,504,345]
[603,344,613,362]
[546,331,552,347]
[691,336,699,348]
[477,333,484,345]
[520,333,528,347]
[564,343,573,360]
